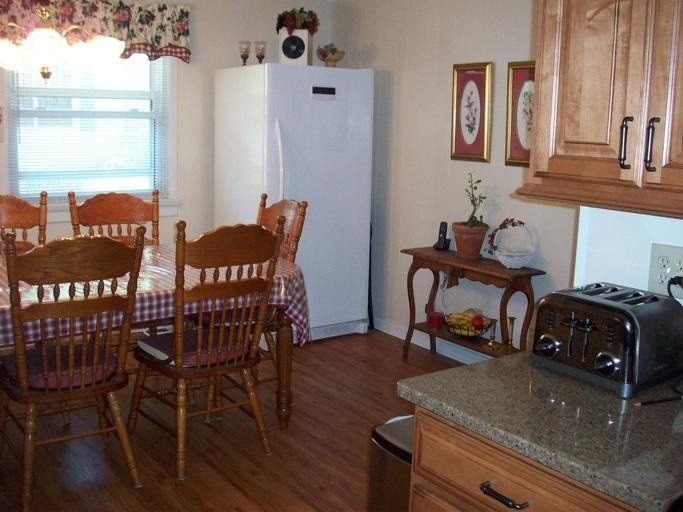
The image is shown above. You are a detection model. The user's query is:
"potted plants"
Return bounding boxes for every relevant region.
[451,171,489,260]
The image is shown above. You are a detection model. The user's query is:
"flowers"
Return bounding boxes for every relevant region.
[483,217,524,255]
[276,7,320,39]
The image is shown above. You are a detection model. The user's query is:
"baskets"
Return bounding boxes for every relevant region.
[441,313,498,337]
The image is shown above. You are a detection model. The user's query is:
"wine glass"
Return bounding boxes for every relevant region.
[239,39,266,65]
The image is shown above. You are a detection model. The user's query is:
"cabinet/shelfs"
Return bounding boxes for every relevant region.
[409,408,638,512]
[532,0,683,194]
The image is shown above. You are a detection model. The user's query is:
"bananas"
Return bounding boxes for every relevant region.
[448,308,476,336]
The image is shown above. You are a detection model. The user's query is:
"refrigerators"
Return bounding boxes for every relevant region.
[212,64,374,345]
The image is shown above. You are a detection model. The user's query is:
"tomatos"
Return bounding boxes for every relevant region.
[473,316,483,330]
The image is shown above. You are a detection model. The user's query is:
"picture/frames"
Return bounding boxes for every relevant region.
[450,60,535,168]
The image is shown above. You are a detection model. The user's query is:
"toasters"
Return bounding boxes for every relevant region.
[531,283,683,401]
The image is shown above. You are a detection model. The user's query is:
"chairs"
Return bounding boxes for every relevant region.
[1,190,71,426]
[202,193,309,424]
[127,214,287,478]
[1,225,147,512]
[67,189,194,353]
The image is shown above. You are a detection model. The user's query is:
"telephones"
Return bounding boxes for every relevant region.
[433,222,450,251]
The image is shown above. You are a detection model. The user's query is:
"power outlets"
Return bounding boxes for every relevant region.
[645,240,683,299]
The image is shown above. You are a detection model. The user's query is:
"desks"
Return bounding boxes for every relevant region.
[2,244,301,426]
[399,244,546,361]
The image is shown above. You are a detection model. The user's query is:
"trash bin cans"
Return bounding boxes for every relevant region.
[367,414,414,512]
